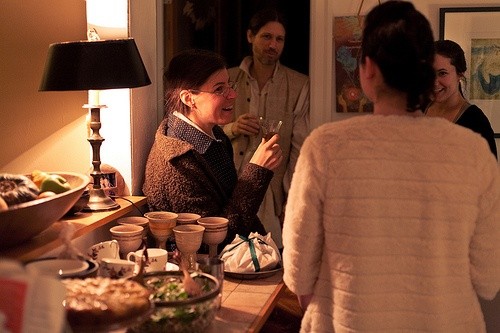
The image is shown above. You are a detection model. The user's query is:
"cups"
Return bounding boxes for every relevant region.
[86,240,122,273]
[100,258,136,279]
[258,120,283,142]
[127,248,168,271]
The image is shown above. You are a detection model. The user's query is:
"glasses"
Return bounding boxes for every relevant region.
[187,79,235,99]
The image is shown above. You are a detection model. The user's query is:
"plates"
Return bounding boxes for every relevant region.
[133,261,179,275]
[224,263,283,278]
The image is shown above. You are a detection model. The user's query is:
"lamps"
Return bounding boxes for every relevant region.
[38,27,153,211]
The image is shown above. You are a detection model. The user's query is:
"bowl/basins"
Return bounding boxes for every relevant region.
[24,256,99,280]
[127,270,220,333]
[69,298,154,333]
[0,171,90,249]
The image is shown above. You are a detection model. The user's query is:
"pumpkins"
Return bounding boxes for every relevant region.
[0,172,40,206]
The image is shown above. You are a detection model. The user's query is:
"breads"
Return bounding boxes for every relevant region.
[56,278,150,331]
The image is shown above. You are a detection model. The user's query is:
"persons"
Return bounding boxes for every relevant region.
[421,40,497,160]
[282,0,500,333]
[216,11,309,256]
[143,48,282,256]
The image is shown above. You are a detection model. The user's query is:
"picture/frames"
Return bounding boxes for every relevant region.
[439,7,500,139]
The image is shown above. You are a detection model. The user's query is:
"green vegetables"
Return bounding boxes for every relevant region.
[140,281,212,333]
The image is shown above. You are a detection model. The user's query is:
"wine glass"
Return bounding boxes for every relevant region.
[110,211,229,268]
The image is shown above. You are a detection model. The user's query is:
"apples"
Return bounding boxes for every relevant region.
[33,172,71,200]
